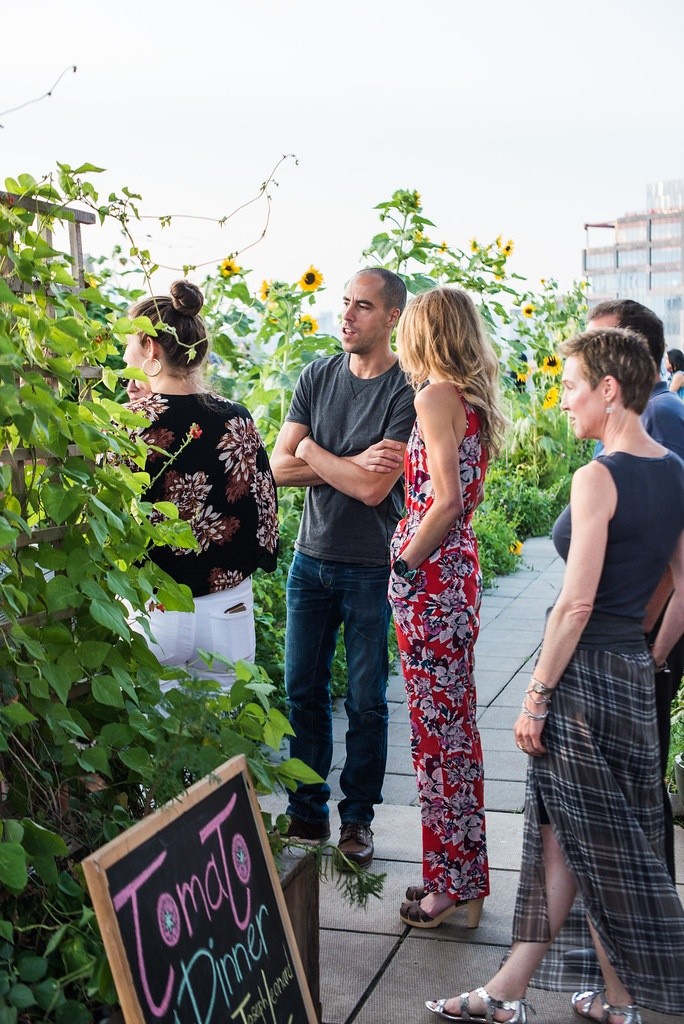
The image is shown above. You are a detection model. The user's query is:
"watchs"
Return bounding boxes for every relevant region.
[392,557,418,579]
[527,674,554,695]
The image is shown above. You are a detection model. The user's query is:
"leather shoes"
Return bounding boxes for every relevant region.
[270,825,331,846]
[334,822,374,868]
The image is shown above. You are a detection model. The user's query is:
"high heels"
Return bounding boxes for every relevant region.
[405,885,427,900]
[400,889,484,927]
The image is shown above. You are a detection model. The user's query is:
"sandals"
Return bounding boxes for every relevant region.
[570,990,643,1024]
[425,987,537,1024]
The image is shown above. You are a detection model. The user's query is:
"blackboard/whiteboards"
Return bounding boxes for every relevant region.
[79,750,320,1024]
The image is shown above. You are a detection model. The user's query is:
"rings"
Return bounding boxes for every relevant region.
[518,744,526,750]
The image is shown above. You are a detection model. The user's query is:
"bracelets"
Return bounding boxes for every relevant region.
[524,690,546,705]
[655,660,668,672]
[520,701,549,720]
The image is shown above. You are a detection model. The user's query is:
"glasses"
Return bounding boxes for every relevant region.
[119,377,135,389]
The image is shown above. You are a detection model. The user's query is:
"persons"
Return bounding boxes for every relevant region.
[664,348,684,403]
[96,276,280,710]
[385,289,509,928]
[588,299,684,888]
[268,269,427,870]
[424,329,684,1024]
